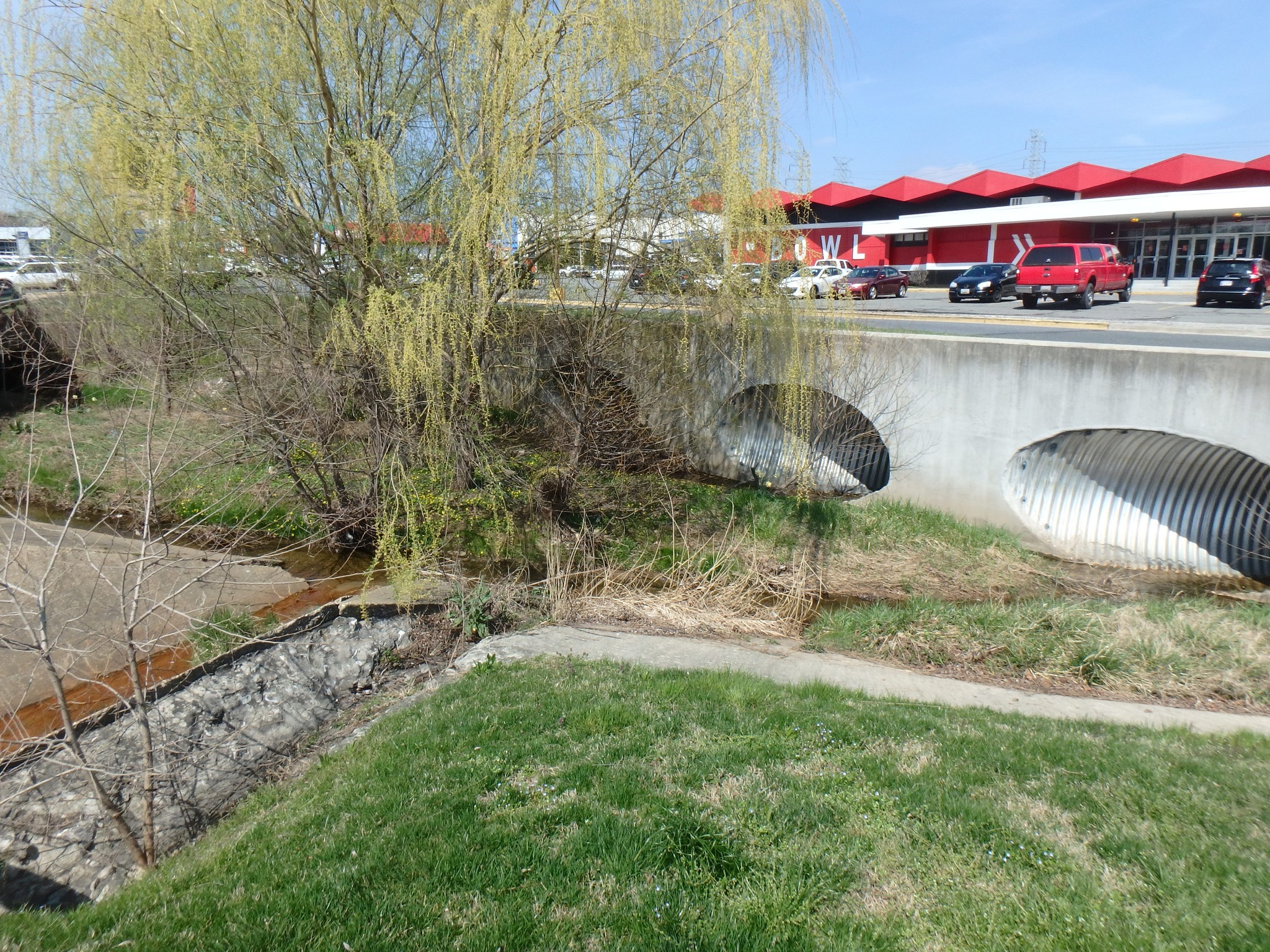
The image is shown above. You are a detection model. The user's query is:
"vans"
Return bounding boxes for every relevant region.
[814,258,857,277]
[776,266,849,299]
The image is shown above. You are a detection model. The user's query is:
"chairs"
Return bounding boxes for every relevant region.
[832,271,838,275]
[812,272,816,276]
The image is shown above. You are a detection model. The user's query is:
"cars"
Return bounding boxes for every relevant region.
[628,260,805,294]
[0,255,75,267]
[168,255,430,293]
[831,266,909,300]
[495,263,535,289]
[949,263,1023,303]
[558,264,630,280]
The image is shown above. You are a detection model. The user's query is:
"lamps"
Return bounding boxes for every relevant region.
[1130,218,1138,222]
[1232,213,1241,217]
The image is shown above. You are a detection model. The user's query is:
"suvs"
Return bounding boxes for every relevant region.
[1195,257,1270,309]
[0,261,84,294]
[1015,243,1137,309]
